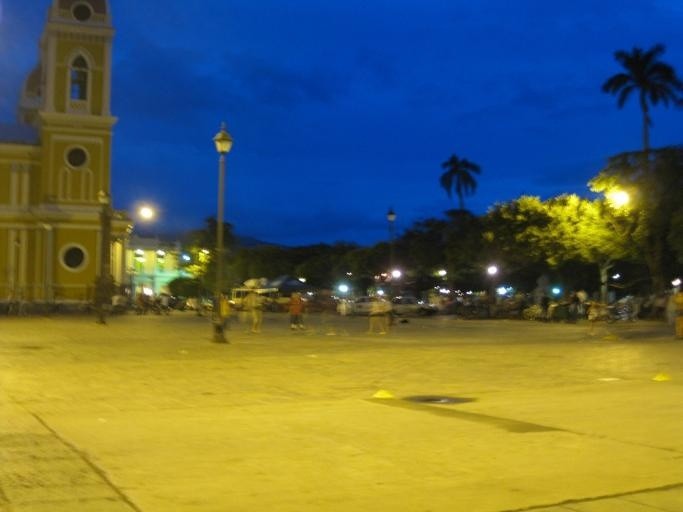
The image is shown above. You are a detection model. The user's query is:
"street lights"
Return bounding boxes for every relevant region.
[95,188,111,325]
[210,118,235,345]
[386,206,397,274]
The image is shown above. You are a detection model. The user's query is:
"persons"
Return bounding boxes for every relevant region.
[539,291,551,323]
[516,297,526,320]
[672,287,683,339]
[571,293,583,321]
[137,289,410,335]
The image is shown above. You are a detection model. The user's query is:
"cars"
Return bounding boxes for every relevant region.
[172,284,340,313]
